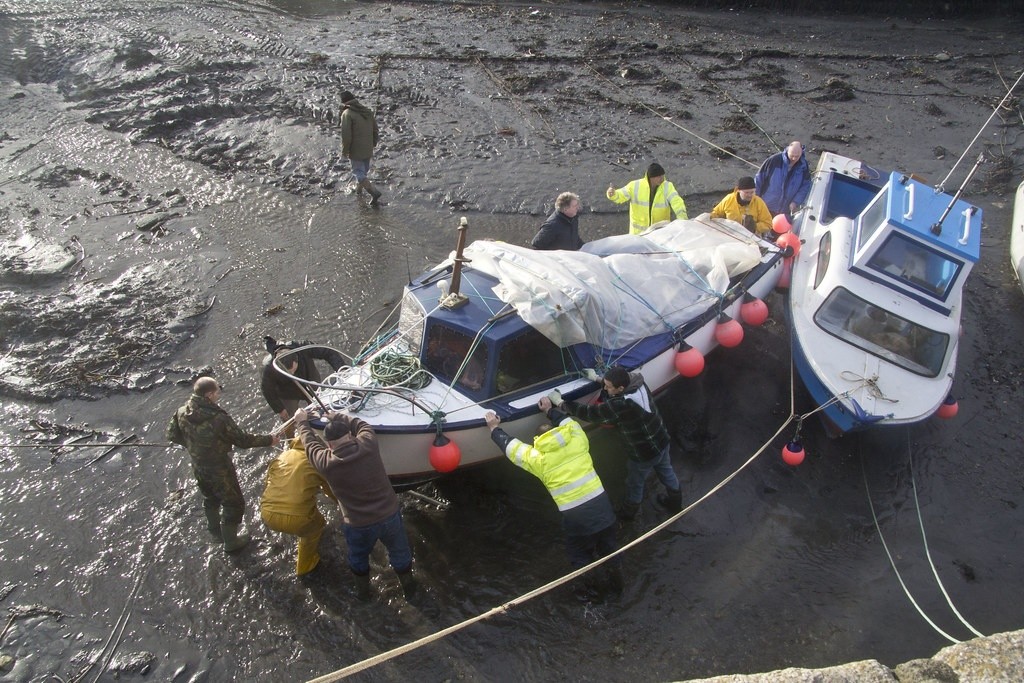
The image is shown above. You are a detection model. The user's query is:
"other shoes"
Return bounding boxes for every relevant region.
[298,560,326,587]
[319,548,342,560]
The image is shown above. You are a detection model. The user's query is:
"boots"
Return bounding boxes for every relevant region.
[580,579,605,603]
[604,568,625,598]
[394,567,419,600]
[204,507,224,542]
[219,521,252,553]
[658,490,683,511]
[353,180,381,205]
[350,567,375,601]
[617,504,639,523]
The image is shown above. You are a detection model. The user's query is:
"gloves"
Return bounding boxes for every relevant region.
[581,368,599,382]
[548,388,563,408]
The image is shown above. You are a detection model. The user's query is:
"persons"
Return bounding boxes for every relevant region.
[531,192,586,251]
[606,163,689,236]
[295,407,417,600]
[483,395,622,605]
[708,176,772,239]
[259,435,339,578]
[165,376,277,554]
[339,91,381,205]
[548,367,682,523]
[754,140,811,245]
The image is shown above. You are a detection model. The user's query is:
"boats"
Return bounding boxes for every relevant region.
[273,211,793,494]
[1009,179,1024,292]
[789,149,983,439]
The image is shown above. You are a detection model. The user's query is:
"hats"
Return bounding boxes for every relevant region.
[647,163,666,178]
[323,421,349,441]
[341,91,355,103]
[738,176,756,190]
